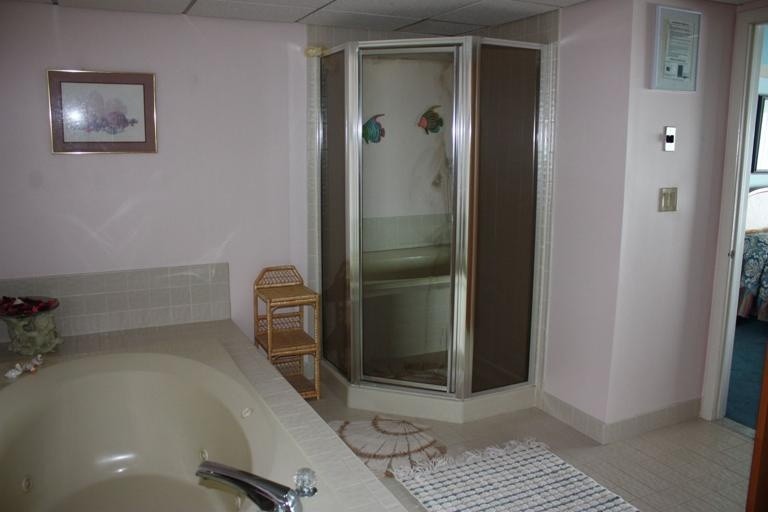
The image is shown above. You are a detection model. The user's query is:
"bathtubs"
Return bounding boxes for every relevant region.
[0,336,346,512]
[362,243,452,361]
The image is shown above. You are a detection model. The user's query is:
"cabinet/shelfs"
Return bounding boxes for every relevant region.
[251,261,324,406]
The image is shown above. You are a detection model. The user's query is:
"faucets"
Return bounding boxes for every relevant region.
[194,459,319,512]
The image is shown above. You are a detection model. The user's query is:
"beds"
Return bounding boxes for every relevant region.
[734,179,767,328]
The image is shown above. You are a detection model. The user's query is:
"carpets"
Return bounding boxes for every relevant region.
[385,433,650,512]
[323,413,450,480]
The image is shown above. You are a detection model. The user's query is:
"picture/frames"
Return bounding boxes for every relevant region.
[45,67,161,156]
[649,2,704,95]
[751,90,767,179]
